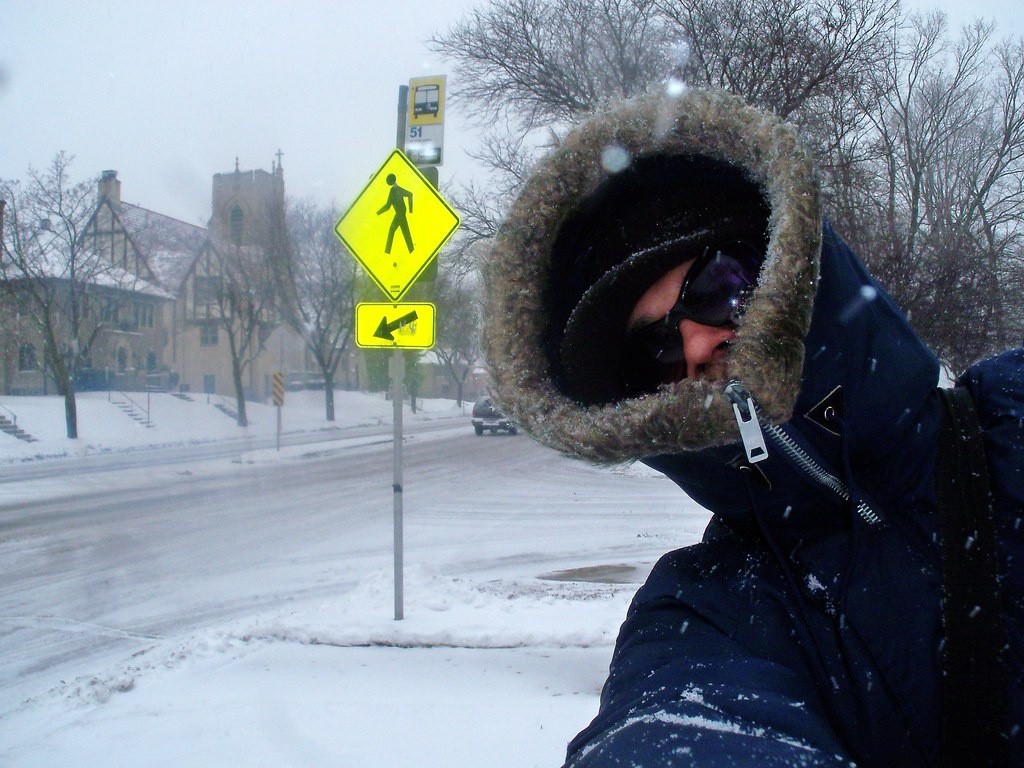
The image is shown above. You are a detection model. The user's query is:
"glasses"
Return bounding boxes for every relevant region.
[608,241,757,401]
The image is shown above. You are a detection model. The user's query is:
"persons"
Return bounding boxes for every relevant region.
[478,91,1024,768]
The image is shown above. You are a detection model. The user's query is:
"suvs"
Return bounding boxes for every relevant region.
[471,395,518,437]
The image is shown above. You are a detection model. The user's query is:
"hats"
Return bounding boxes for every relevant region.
[563,202,748,380]
